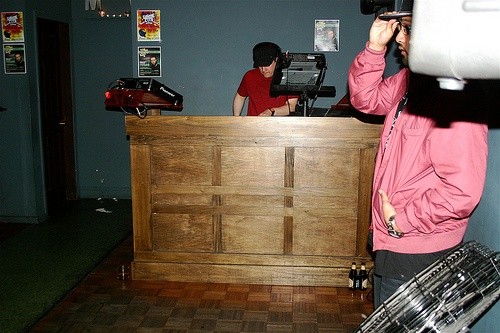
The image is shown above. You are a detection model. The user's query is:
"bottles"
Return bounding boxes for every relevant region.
[358,262,369,291]
[348,262,358,291]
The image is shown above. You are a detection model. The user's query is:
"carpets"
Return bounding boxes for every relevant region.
[0,199,132,333]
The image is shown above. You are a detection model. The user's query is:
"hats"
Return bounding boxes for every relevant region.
[253,42,281,68]
[379,0,414,22]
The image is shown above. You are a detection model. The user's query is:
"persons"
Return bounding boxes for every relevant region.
[145,56,160,76]
[232,41,300,116]
[348,0,488,310]
[14,53,25,72]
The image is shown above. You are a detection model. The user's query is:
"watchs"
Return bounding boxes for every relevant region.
[387,215,403,238]
[269,108,275,116]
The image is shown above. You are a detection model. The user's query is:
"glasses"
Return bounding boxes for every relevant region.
[397,23,411,35]
